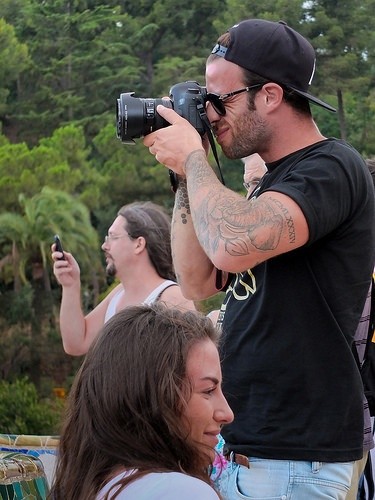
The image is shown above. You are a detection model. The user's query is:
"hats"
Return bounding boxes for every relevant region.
[212,19,338,112]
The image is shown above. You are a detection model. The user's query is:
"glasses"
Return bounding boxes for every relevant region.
[105,235,148,248]
[243,181,260,191]
[205,81,295,116]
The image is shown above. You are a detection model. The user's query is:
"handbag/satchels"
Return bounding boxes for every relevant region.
[362,342,375,416]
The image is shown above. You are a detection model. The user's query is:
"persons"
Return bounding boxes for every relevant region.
[238,145,375,500]
[49,199,199,358]
[141,18,375,500]
[52,303,236,500]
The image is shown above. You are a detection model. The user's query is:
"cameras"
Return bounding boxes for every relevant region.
[115,81,206,145]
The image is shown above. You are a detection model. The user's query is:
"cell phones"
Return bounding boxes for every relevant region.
[54,235,65,260]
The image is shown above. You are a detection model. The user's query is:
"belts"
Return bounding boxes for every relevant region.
[225,451,250,469]
